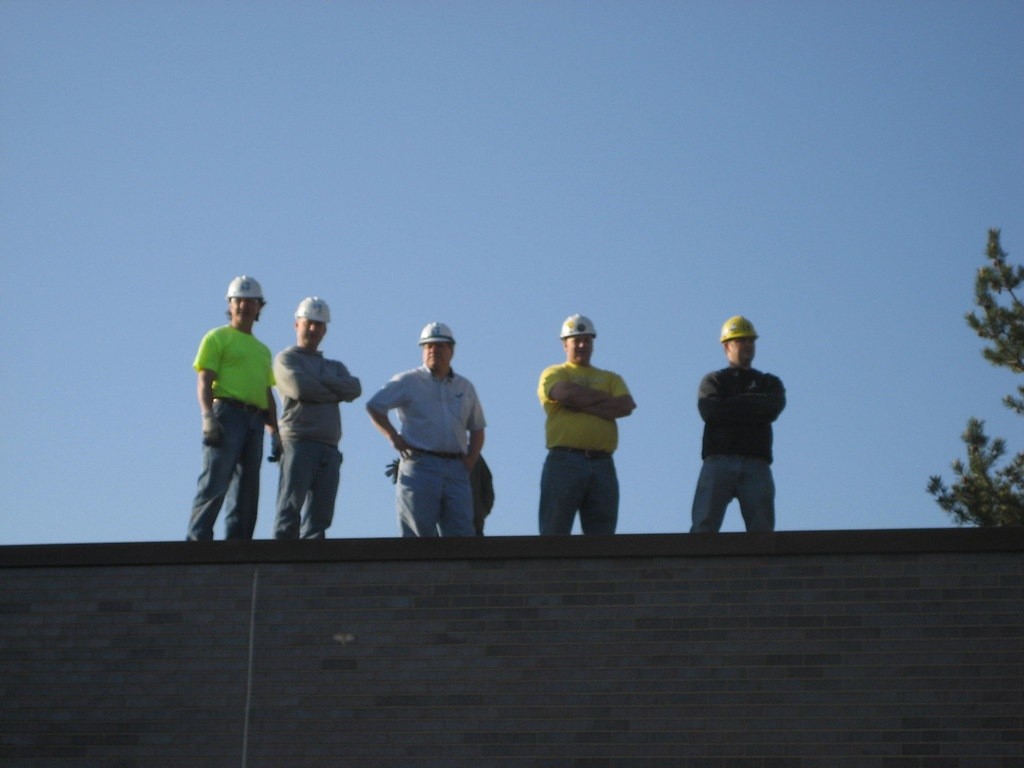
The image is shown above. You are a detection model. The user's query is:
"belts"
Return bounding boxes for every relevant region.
[214,398,265,417]
[412,447,462,460]
[558,447,611,458]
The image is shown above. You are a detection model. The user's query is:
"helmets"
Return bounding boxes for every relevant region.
[721,314,756,343]
[228,275,262,298]
[559,314,596,339]
[417,321,454,345]
[295,297,331,323]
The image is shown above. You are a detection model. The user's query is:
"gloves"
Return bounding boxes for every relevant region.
[268,432,284,463]
[385,457,400,483]
[201,411,224,447]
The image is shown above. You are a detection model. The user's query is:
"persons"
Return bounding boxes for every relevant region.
[186,275,283,541]
[538,314,636,535]
[689,315,786,532]
[273,297,362,539]
[365,320,487,538]
